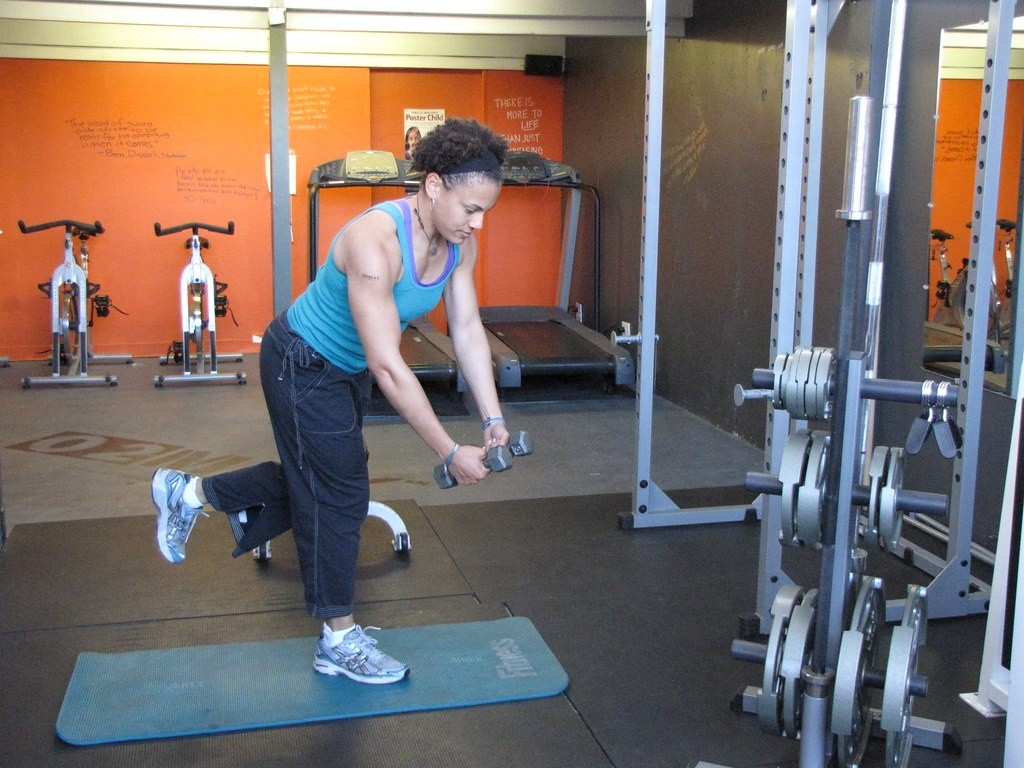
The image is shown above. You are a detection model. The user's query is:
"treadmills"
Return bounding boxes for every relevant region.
[306,147,636,404]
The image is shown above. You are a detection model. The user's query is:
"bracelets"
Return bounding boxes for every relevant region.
[445,443,460,466]
[481,417,505,430]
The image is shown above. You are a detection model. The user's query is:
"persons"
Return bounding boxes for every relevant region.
[151,117,529,685]
[404,127,422,160]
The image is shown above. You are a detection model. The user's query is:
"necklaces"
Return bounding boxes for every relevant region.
[414,193,438,256]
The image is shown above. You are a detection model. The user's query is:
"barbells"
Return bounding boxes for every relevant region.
[508,431,535,457]
[434,445,513,490]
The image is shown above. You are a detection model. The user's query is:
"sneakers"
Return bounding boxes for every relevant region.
[151,468,210,564]
[312,621,410,684]
